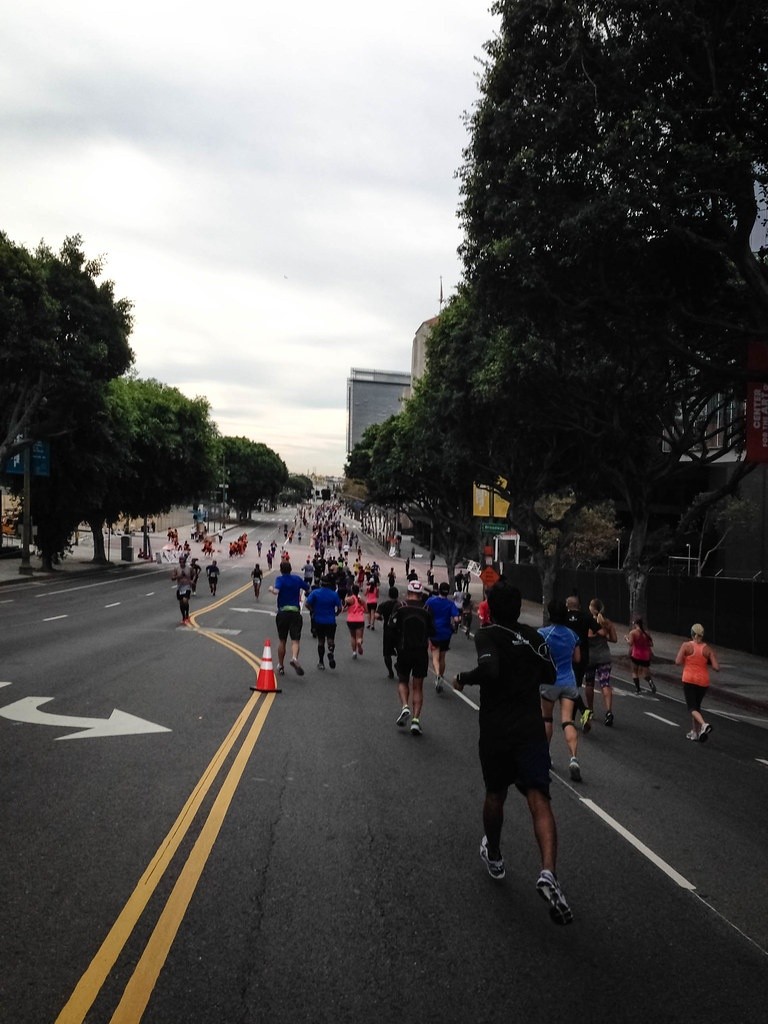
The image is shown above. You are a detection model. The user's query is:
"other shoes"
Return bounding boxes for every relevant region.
[289,660,304,675]
[327,653,336,668]
[358,642,363,655]
[435,675,444,693]
[651,684,656,693]
[317,662,325,669]
[182,618,190,623]
[367,625,374,630]
[277,665,284,674]
[352,653,357,658]
[635,689,641,694]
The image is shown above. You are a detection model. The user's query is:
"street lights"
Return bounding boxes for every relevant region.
[18,384,48,576]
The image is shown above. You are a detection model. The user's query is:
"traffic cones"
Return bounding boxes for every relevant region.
[249,640,282,693]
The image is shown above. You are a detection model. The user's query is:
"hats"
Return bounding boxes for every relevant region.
[408,581,423,593]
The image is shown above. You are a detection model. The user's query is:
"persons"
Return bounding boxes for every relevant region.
[167,527,178,545]
[624,619,658,694]
[218,528,223,543]
[229,533,248,559]
[171,557,197,625]
[673,624,720,741]
[267,540,491,691]
[283,502,362,560]
[536,594,618,782]
[206,560,220,596]
[451,583,574,929]
[204,540,214,557]
[249,563,263,601]
[256,541,262,557]
[183,541,191,559]
[190,527,196,540]
[152,521,155,533]
[190,559,201,595]
[387,580,435,736]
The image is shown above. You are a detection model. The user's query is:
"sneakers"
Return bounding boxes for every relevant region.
[605,713,614,726]
[536,869,574,926]
[396,706,411,727]
[479,836,505,879]
[699,723,712,742]
[568,758,581,781]
[686,731,698,741]
[581,709,593,733]
[410,721,424,735]
